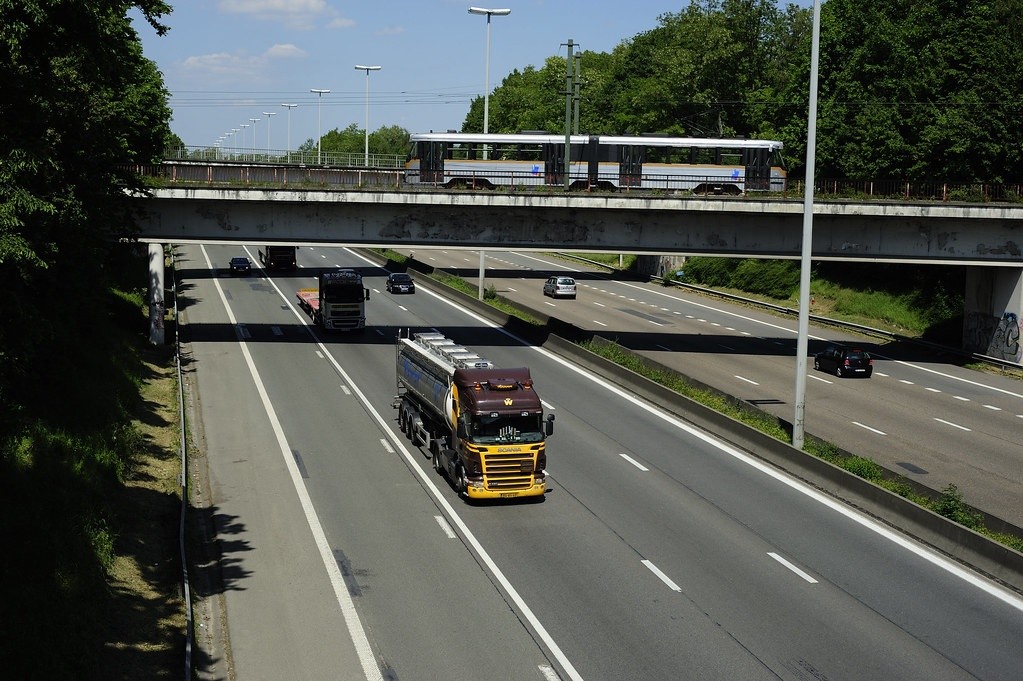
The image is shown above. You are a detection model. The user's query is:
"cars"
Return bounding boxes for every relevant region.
[543,276,578,299]
[387,273,415,294]
[814,346,873,378]
[228,257,253,277]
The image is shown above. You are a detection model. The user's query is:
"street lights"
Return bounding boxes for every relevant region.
[240,124,250,161]
[263,112,276,163]
[231,128,241,161]
[281,103,298,163]
[354,65,383,166]
[249,118,261,162]
[310,88,331,164]
[466,6,511,161]
[213,132,235,160]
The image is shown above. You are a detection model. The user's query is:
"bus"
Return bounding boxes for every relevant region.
[401,130,789,196]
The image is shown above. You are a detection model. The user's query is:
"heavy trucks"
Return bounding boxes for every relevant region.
[396,326,555,501]
[296,268,370,331]
[258,246,299,274]
